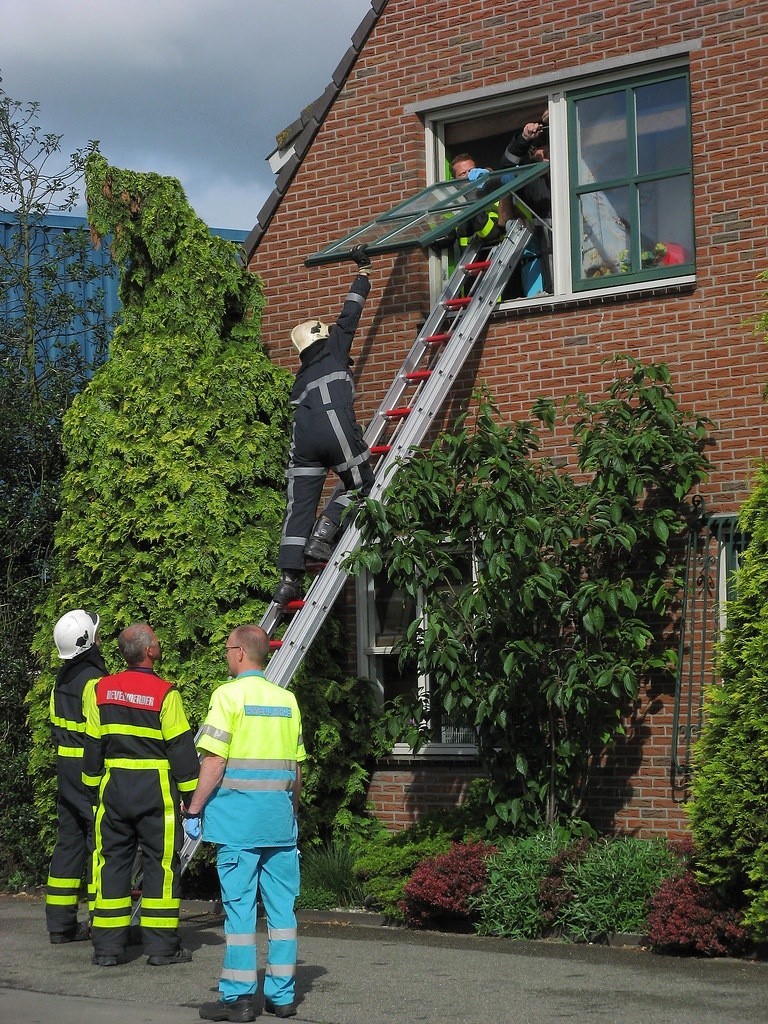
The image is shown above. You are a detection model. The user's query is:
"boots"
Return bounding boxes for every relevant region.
[304,515,339,562]
[271,569,307,606]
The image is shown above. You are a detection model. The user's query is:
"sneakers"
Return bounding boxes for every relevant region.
[196,995,256,1022]
[89,952,119,967]
[144,946,192,967]
[47,922,91,943]
[261,999,298,1019]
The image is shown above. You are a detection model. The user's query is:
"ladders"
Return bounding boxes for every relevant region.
[128,218,534,928]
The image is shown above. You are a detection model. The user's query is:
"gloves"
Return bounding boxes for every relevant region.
[347,243,373,275]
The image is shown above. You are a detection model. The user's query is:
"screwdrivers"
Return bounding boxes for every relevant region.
[537,125,549,131]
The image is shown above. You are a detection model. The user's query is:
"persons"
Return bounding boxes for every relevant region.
[272,243,376,607]
[44,609,143,946]
[449,153,525,303]
[81,622,202,966]
[183,624,307,1023]
[497,108,553,295]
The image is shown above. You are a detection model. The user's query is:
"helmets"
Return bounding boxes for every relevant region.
[289,319,331,354]
[53,608,100,661]
